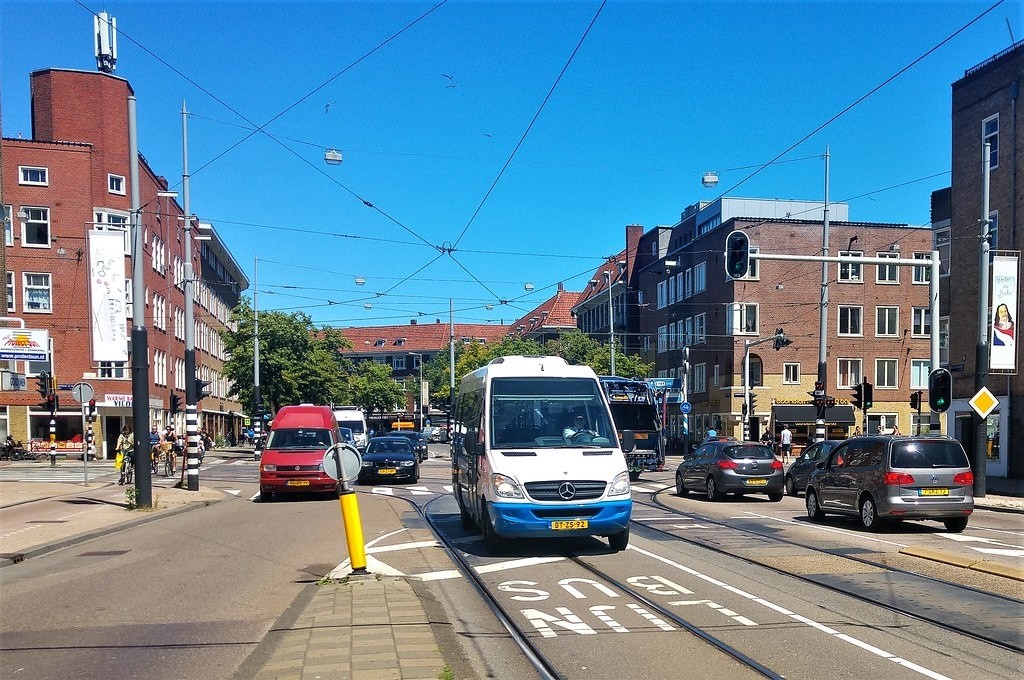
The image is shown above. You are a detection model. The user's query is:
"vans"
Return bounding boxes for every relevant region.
[259,403,343,502]
[330,406,367,451]
[804,434,974,534]
[451,354,632,550]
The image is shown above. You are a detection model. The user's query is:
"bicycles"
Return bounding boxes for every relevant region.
[116,448,134,486]
[149,441,178,477]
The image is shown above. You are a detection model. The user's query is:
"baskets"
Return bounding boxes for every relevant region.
[161,443,172,450]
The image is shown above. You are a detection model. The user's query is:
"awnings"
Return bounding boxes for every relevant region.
[774,406,856,426]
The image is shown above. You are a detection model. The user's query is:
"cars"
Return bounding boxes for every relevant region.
[427,428,449,444]
[359,430,428,485]
[783,438,848,498]
[675,436,785,502]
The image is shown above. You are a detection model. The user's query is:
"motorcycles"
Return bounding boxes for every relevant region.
[0,434,23,461]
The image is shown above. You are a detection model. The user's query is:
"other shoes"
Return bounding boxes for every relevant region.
[172,465,176,472]
[151,469,155,473]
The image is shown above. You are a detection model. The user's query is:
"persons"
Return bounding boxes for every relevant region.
[707,425,721,436]
[562,414,600,445]
[283,433,293,446]
[892,425,899,435]
[150,425,164,465]
[163,425,178,471]
[261,430,267,436]
[760,424,793,464]
[115,424,134,481]
[85,426,96,460]
[239,429,255,446]
[855,426,861,435]
[197,427,212,465]
[5,435,23,460]
[353,425,363,432]
[878,426,884,435]
[368,428,374,438]
[310,432,328,446]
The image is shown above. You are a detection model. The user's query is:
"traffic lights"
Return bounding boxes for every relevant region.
[195,379,213,402]
[169,388,184,414]
[928,368,951,414]
[34,370,48,398]
[725,232,751,279]
[849,384,873,411]
[88,399,96,415]
[48,394,56,412]
[750,392,758,414]
[807,389,819,408]
[910,393,918,409]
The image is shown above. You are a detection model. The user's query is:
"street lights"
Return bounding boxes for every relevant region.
[252,252,366,413]
[525,277,616,377]
[408,351,423,430]
[178,98,342,492]
[698,143,832,446]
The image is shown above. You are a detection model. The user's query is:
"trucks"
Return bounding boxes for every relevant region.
[598,375,666,481]
